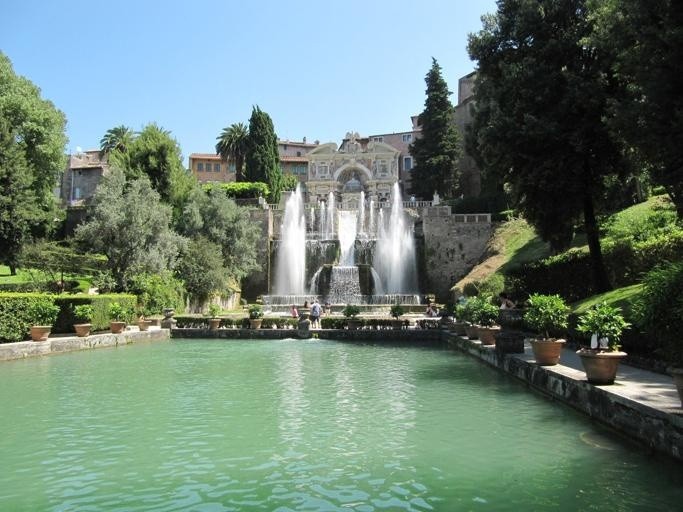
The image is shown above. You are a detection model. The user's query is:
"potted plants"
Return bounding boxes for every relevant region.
[389,303,405,331]
[343,305,361,330]
[207,301,222,329]
[450,259,683,407]
[247,304,266,328]
[28,300,162,342]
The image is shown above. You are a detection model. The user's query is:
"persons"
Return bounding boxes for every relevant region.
[498,291,516,311]
[304,300,307,308]
[325,300,333,316]
[292,305,299,328]
[313,299,321,329]
[309,301,314,328]
